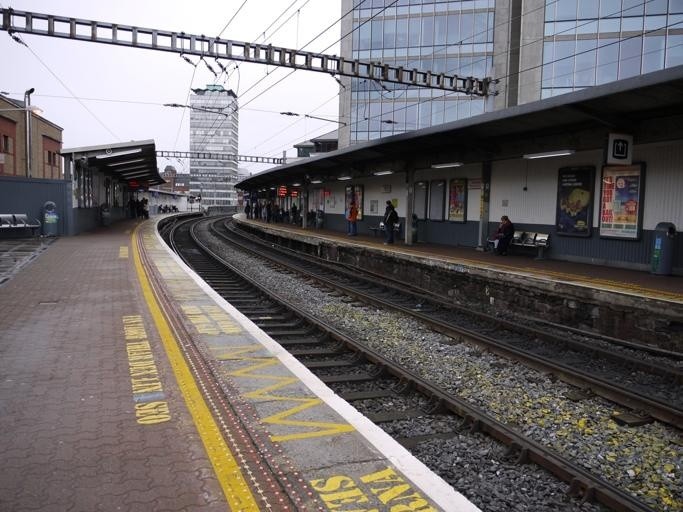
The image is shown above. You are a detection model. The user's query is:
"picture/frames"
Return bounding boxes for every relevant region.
[412,178,467,224]
[555,161,647,242]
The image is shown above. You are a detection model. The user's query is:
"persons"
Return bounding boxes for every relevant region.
[382,200,399,245]
[242,199,323,229]
[488,215,513,255]
[344,204,349,234]
[346,200,357,235]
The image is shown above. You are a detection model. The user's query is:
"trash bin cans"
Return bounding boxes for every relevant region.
[650,222,676,276]
[42,201,59,236]
[411,213,418,243]
[316,209,324,230]
[101,208,112,226]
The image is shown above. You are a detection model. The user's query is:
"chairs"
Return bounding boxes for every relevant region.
[510,231,550,261]
[367,221,401,241]
[0,213,41,237]
